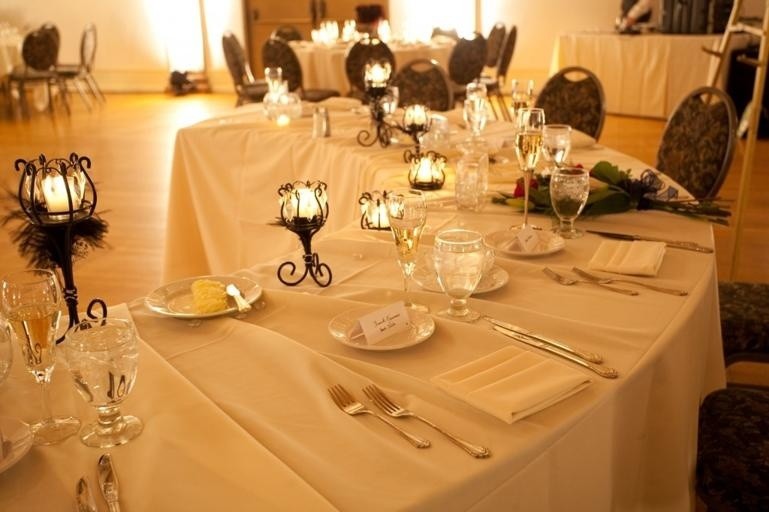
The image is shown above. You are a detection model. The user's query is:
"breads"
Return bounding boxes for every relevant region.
[190,279,228,314]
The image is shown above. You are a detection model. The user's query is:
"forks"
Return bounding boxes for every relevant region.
[326,383,432,450]
[571,266,687,297]
[361,381,492,459]
[542,266,638,298]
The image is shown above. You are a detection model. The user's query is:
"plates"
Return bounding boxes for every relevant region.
[410,261,511,295]
[0,413,35,476]
[483,228,565,256]
[325,302,436,353]
[142,273,263,319]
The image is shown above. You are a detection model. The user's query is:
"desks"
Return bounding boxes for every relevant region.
[550,31,750,121]
[1,103,728,511]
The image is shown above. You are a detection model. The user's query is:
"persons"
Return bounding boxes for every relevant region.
[623,0,688,33]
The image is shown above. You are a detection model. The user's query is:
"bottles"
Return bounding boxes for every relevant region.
[308,107,331,138]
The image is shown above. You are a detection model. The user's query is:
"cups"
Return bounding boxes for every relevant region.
[452,150,489,212]
[613,16,625,34]
[262,67,302,122]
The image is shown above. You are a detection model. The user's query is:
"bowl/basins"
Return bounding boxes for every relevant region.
[480,246,495,276]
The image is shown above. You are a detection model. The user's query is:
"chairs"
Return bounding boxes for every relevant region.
[0,20,106,121]
[530,67,607,140]
[699,386,768,511]
[653,83,738,208]
[718,279,769,369]
[221,24,517,120]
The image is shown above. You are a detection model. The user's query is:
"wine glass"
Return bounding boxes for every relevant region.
[509,107,591,239]
[0,266,81,446]
[431,230,483,322]
[61,317,144,450]
[375,76,533,157]
[383,187,430,314]
[308,16,391,51]
[0,307,16,464]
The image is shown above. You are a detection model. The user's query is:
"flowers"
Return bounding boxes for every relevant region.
[491,161,734,220]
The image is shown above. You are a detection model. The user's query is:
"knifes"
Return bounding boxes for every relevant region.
[482,314,604,364]
[223,282,253,315]
[95,451,122,511]
[73,472,97,511]
[491,325,618,379]
[585,230,714,255]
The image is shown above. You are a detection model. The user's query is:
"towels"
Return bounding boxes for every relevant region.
[587,237,667,276]
[431,344,592,427]
